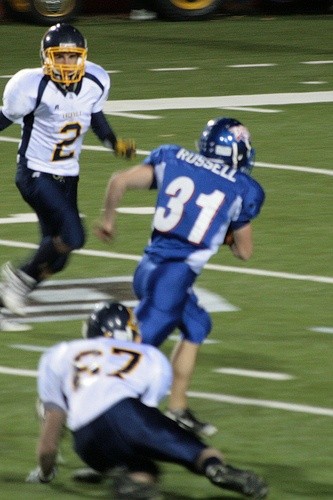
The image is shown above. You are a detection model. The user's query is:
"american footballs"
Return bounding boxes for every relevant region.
[223,227,235,245]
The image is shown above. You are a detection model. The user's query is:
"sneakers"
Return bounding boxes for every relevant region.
[207,463,268,499]
[111,475,159,500]
[0,262,36,317]
[164,408,217,436]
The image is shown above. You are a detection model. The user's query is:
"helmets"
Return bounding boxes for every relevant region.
[198,117,254,173]
[83,301,141,344]
[40,22,87,83]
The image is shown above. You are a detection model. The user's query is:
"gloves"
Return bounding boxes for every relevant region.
[25,466,59,484]
[116,138,136,157]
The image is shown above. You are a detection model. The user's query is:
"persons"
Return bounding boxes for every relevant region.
[93,117,266,436]
[0,21,137,331]
[26,299,270,500]
[129,0,157,20]
